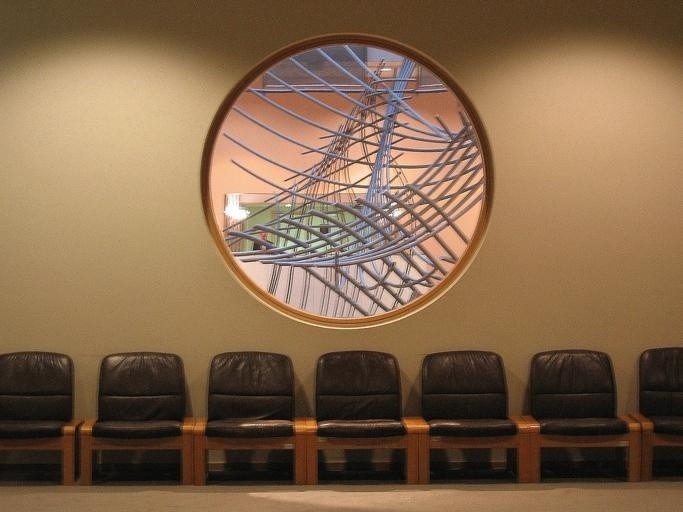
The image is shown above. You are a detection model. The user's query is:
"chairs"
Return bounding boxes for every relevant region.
[307,349,419,486]
[194,350,307,487]
[0,351,83,486]
[520,348,642,483]
[78,352,196,487]
[418,350,533,485]
[626,346,682,481]
